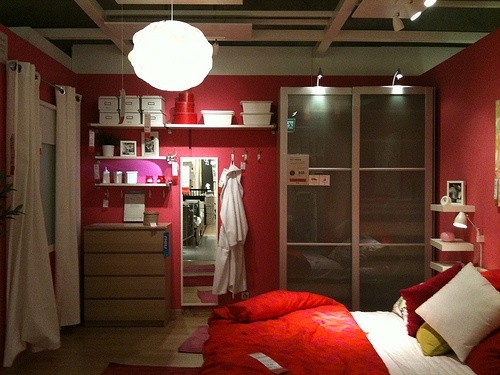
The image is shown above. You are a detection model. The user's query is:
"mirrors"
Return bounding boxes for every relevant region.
[180,157,218,306]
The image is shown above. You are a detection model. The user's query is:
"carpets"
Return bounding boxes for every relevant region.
[178,326,209,353]
[101,362,201,375]
[184,264,217,303]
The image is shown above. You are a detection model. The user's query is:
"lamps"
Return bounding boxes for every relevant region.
[392,68,404,85]
[127,0,214,92]
[317,68,323,86]
[453,212,488,272]
[393,0,437,32]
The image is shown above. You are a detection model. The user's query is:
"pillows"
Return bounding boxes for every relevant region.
[392,262,500,368]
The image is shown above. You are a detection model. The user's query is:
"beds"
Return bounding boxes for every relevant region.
[201,267,500,375]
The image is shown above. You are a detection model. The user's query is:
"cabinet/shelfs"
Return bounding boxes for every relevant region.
[83,223,172,327]
[91,124,276,199]
[430,204,475,272]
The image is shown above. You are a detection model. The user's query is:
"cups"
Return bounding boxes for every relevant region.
[124,171,138,183]
[144,212,158,225]
[102,145,115,156]
[113,171,122,183]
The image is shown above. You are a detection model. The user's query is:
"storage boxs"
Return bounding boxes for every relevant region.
[201,109,235,125]
[240,101,273,125]
[99,96,167,124]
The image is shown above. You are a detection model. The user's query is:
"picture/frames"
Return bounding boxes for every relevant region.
[120,132,160,157]
[447,180,467,205]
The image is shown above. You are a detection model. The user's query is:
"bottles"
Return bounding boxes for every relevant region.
[146,175,166,183]
[102,167,110,183]
